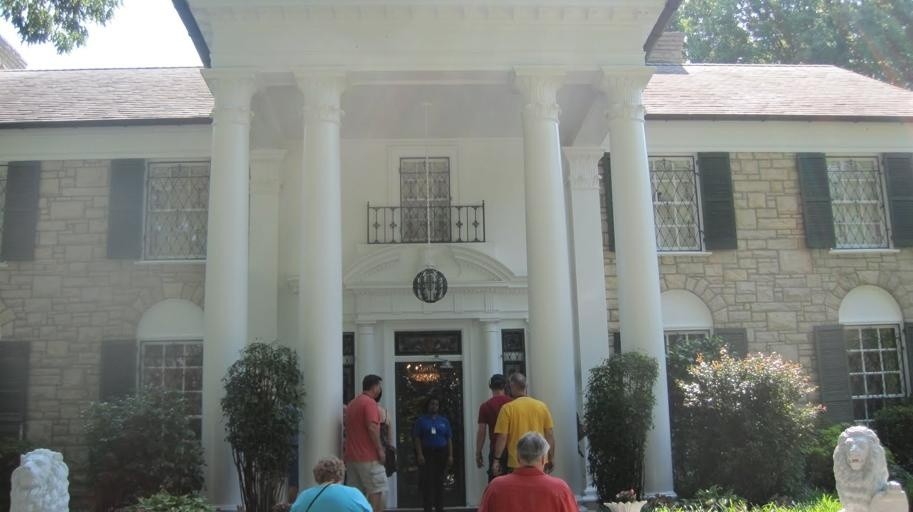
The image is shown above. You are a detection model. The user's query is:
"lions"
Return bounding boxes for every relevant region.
[832,425,889,512]
[10,448,71,512]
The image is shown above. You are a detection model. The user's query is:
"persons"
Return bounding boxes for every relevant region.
[370,383,394,466]
[342,373,390,512]
[486,371,555,483]
[288,455,373,511]
[472,373,514,481]
[493,453,502,461]
[472,431,580,512]
[411,394,454,512]
